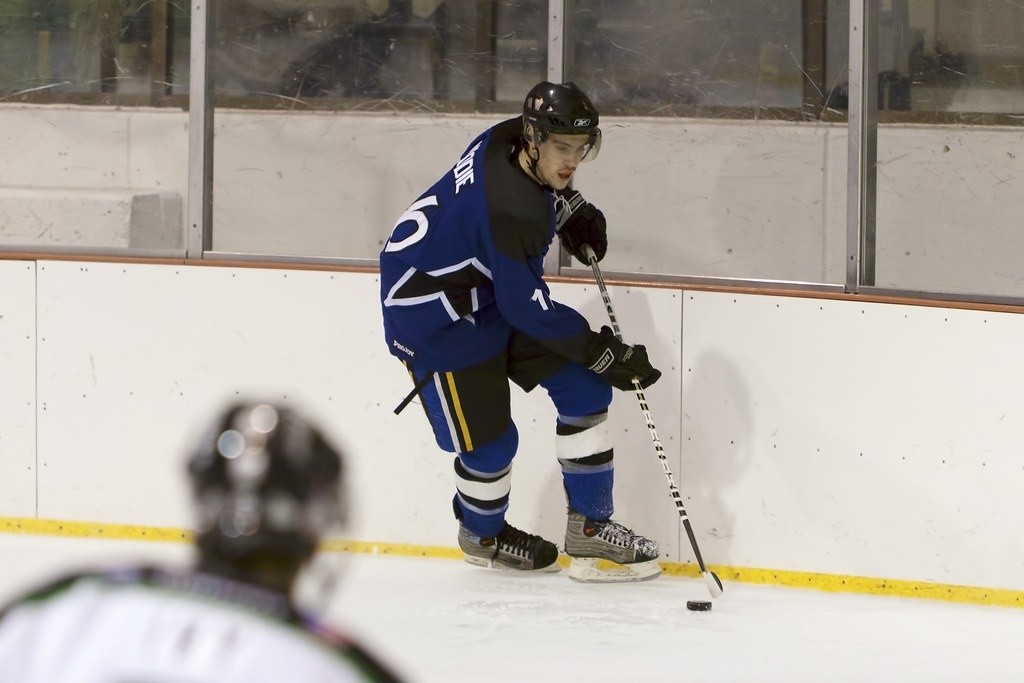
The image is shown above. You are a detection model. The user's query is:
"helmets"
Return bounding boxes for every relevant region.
[190,405,341,550]
[523,81,599,146]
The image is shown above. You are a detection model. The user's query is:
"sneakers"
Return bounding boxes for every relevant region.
[458,519,561,574]
[565,507,662,583]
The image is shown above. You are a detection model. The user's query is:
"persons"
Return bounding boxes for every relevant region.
[379,81,663,582]
[1,401,403,683]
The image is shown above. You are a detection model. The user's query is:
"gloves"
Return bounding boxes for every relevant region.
[551,186,607,266]
[586,326,661,391]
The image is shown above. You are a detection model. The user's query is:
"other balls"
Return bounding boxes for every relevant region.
[687,600,712,612]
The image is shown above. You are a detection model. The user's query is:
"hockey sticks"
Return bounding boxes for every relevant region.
[584,244,725,598]
